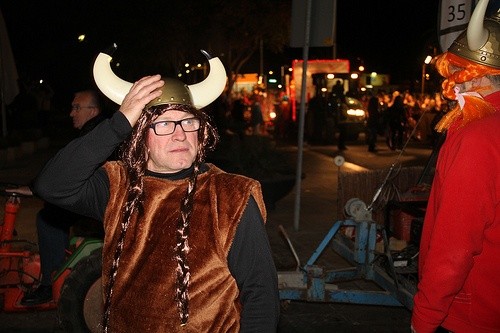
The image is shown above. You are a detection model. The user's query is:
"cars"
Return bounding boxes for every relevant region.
[308,93,368,140]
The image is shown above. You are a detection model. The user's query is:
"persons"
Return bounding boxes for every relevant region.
[5,88,124,305]
[367,96,409,152]
[35,74,279,333]
[333,81,344,98]
[411,20,500,333]
[234,95,262,134]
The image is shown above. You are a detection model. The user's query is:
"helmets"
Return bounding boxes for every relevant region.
[93,42,227,110]
[447,0,500,69]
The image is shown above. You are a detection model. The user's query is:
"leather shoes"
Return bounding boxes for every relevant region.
[21,285,53,307]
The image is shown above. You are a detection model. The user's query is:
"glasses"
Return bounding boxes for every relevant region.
[71,104,96,112]
[147,116,201,135]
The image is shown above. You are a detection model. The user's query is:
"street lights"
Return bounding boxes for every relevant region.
[421,55,433,96]
